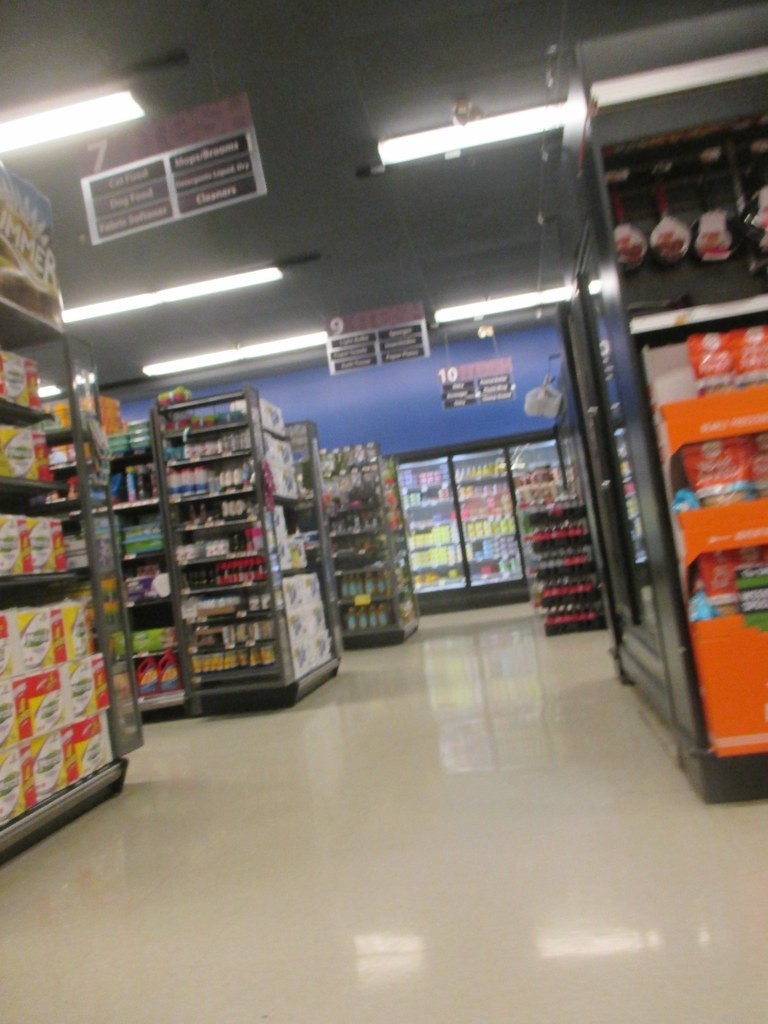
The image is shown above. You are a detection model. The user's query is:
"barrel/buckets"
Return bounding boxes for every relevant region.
[134,648,182,700]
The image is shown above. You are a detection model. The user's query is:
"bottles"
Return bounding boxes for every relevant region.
[527,524,587,552]
[339,570,391,599]
[541,576,594,606]
[545,603,599,636]
[343,602,391,630]
[455,464,500,478]
[529,494,587,525]
[537,549,593,577]
[167,467,208,496]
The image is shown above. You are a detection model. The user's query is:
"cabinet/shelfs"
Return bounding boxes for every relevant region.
[0,161,153,862]
[104,387,346,717]
[528,498,607,637]
[319,440,420,650]
